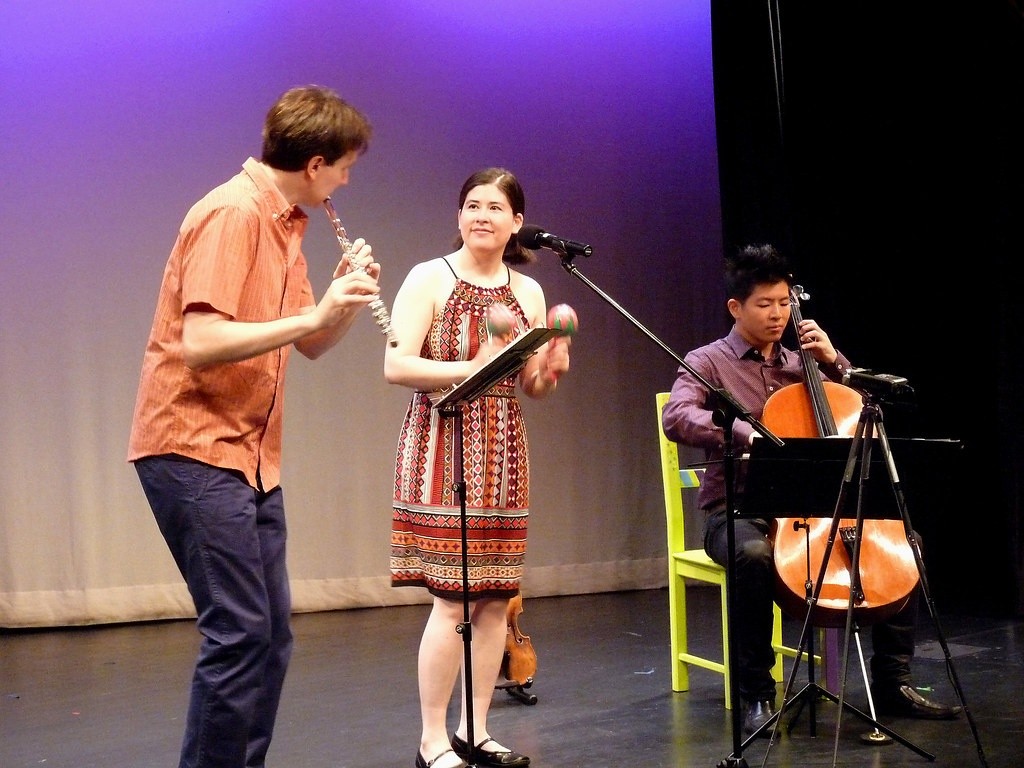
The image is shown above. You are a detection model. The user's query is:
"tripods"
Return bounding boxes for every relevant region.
[717,394,989,768]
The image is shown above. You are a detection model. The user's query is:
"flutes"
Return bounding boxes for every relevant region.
[321,192,396,349]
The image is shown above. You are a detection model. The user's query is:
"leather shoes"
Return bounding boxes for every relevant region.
[451,732,530,767]
[415,748,477,768]
[744,698,782,739]
[867,682,962,721]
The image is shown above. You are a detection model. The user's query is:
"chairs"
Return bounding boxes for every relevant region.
[656,392,842,709]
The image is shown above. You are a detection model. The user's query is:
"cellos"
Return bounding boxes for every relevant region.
[744,267,925,741]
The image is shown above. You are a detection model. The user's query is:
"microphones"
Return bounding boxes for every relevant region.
[847,369,908,394]
[518,225,592,258]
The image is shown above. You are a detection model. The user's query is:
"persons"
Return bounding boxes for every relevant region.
[125,85,381,768]
[661,247,963,739]
[384,165,570,768]
